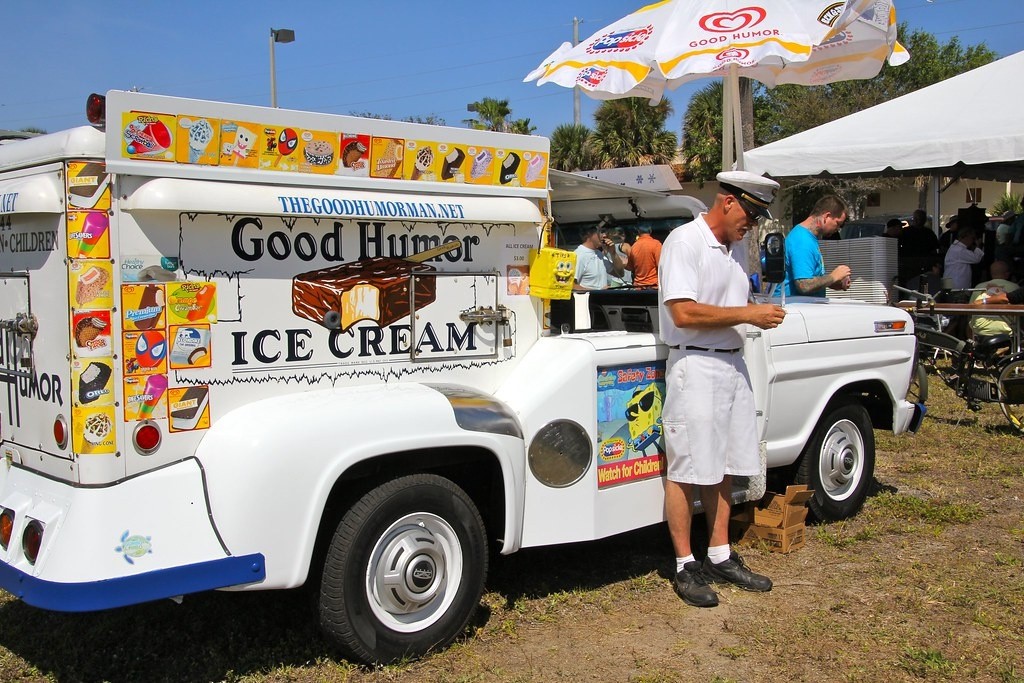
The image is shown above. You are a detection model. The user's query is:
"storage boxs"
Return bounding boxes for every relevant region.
[749,484,815,528]
[729,507,809,554]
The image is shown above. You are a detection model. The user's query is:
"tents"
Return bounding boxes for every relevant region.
[721,42,1023,185]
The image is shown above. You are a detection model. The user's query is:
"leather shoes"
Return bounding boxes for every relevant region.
[673,561,719,606]
[703,551,773,592]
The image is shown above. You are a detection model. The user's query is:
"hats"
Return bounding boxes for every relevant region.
[716,171,780,220]
[887,219,906,228]
[946,215,958,228]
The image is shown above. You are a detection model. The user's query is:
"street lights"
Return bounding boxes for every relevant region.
[268,26,297,109]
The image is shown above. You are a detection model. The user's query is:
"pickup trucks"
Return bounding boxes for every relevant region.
[0,91,927,669]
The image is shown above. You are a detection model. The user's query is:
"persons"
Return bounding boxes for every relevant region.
[782,193,851,298]
[571,221,663,292]
[657,169,787,609]
[882,195,1024,340]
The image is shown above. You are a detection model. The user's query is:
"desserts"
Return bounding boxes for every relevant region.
[303,140,334,165]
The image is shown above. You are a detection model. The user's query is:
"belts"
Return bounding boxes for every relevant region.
[669,344,739,353]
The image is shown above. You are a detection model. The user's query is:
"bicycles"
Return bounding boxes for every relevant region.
[913,325,1024,435]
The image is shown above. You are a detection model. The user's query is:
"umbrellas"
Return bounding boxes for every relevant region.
[521,0,912,170]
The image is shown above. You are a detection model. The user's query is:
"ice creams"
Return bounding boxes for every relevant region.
[188,118,214,162]
[80,412,112,454]
[410,146,434,181]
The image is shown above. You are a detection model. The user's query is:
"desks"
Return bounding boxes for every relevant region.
[893,302,1024,375]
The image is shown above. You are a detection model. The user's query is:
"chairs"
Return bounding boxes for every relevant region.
[899,300,952,360]
[971,297,1016,370]
